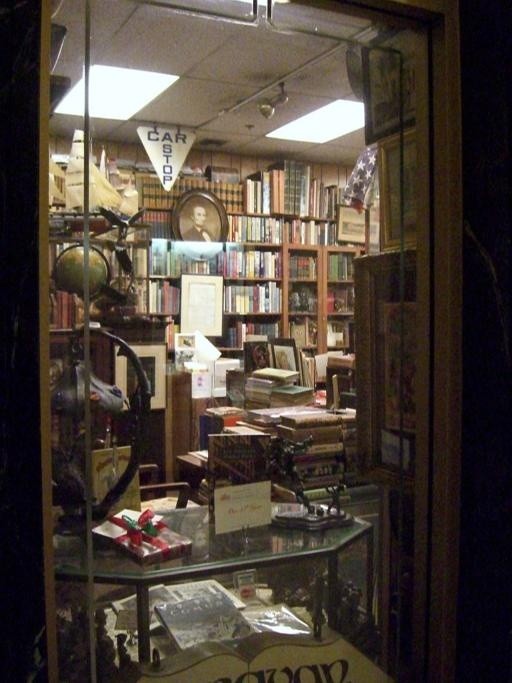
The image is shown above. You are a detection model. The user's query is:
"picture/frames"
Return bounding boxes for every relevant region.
[362,26,419,146]
[351,248,418,494]
[110,340,168,412]
[179,271,225,338]
[169,186,229,260]
[376,125,419,251]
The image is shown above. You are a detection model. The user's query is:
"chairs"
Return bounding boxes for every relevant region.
[138,461,202,535]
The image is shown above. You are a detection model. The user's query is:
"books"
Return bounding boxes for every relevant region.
[327,253,355,347]
[56,159,380,248]
[131,248,283,347]
[288,253,318,348]
[110,583,180,638]
[164,579,246,611]
[237,601,314,638]
[50,241,130,328]
[203,350,360,536]
[153,590,254,653]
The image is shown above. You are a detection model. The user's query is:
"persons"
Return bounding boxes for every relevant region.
[181,204,217,241]
[274,350,292,371]
[251,344,268,370]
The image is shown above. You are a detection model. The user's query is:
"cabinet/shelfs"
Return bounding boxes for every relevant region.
[52,497,376,682]
[286,245,357,360]
[52,244,286,380]
